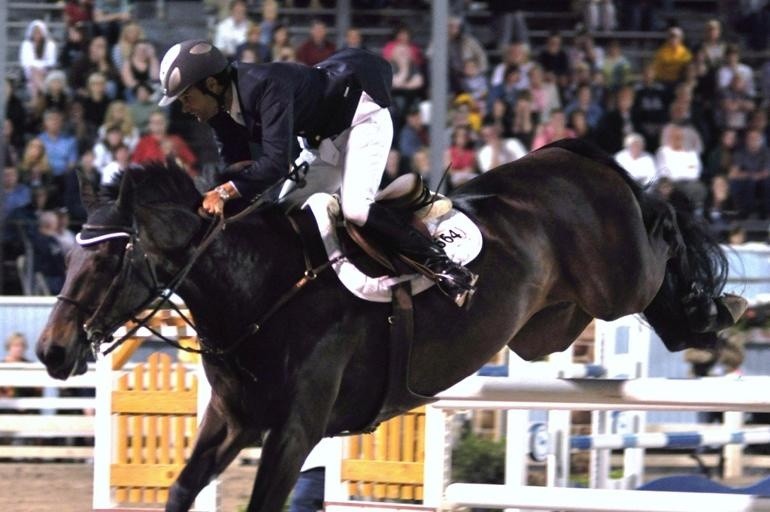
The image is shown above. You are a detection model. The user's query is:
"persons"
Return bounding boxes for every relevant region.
[0,0,166,296]
[216,2,770,245]
[0,333,96,447]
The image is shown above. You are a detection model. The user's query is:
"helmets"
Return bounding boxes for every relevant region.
[159,40,229,107]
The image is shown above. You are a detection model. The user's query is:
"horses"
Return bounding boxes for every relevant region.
[31,133,751,510]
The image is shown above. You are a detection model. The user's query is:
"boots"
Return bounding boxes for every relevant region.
[360,203,471,295]
